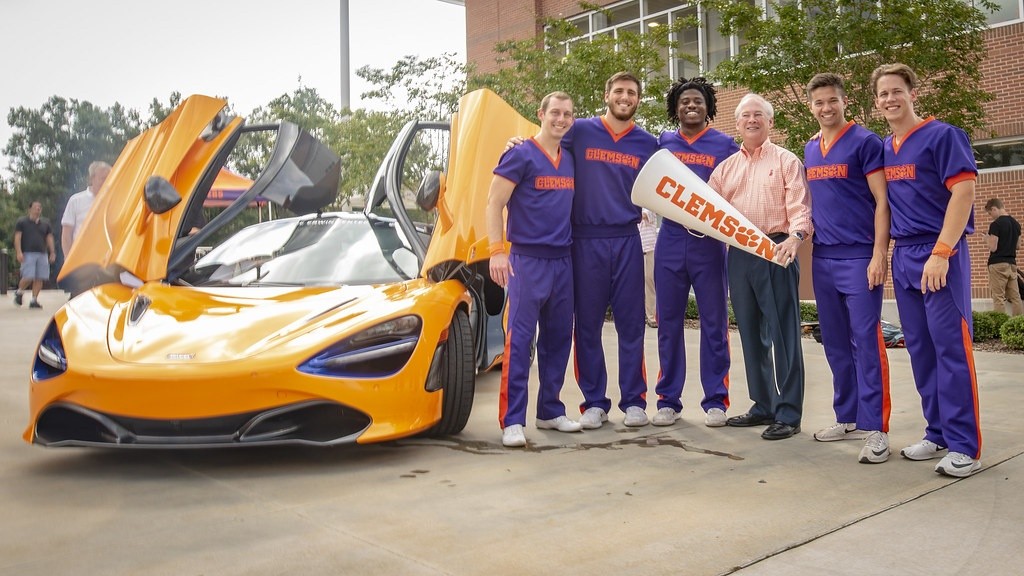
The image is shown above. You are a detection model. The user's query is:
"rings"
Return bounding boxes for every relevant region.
[787,252,789,253]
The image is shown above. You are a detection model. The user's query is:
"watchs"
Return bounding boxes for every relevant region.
[791,232,804,243]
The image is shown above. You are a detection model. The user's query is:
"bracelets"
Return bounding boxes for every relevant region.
[489,241,504,255]
[932,241,952,259]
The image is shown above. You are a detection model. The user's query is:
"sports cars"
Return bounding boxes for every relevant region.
[23,87,540,449]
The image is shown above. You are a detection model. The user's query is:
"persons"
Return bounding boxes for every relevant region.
[504,71,656,426]
[649,78,739,427]
[805,72,892,463]
[870,62,982,477]
[62,161,111,259]
[186,209,205,236]
[982,198,1024,317]
[708,95,813,439]
[486,90,582,445]
[14,200,55,309]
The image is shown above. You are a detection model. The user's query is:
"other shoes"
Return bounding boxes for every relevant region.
[29,301,43,308]
[14,290,22,304]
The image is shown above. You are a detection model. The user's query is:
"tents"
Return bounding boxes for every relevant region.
[204,168,272,222]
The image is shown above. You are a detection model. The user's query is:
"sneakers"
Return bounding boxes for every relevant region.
[653,406,681,425]
[623,406,648,426]
[535,414,582,432]
[705,408,727,426]
[857,431,889,463]
[814,423,875,442]
[579,406,608,428]
[900,440,950,461]
[934,451,982,477]
[502,424,526,447]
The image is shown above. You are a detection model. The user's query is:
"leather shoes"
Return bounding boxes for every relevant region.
[761,420,801,438]
[726,412,775,426]
